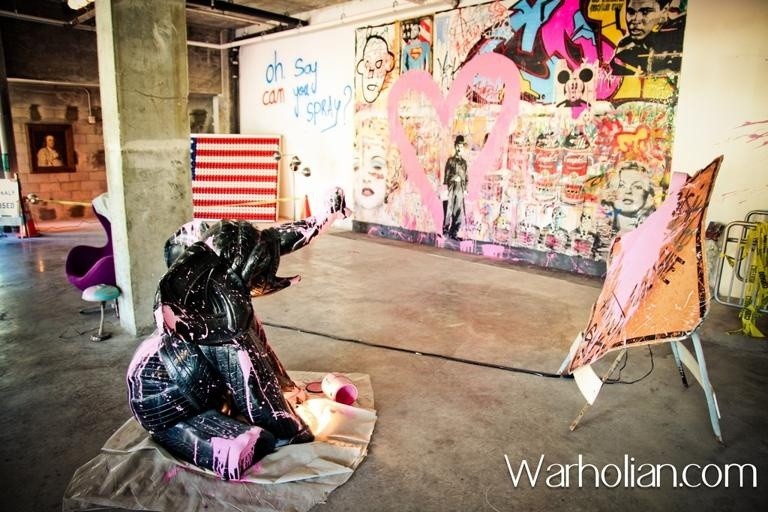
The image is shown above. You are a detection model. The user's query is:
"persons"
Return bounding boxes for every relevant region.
[356,117,405,224]
[37,134,63,167]
[610,0,680,74]
[401,22,429,74]
[608,161,659,261]
[440,135,469,240]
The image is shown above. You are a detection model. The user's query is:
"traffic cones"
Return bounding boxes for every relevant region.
[15,196,43,239]
[298,194,311,220]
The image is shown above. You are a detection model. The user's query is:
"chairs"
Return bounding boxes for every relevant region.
[64,191,120,319]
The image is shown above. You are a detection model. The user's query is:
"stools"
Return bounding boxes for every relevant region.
[82,284,120,342]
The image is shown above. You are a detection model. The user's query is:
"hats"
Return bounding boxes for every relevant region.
[454,135,467,144]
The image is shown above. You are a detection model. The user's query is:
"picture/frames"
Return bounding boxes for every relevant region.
[24,121,77,174]
